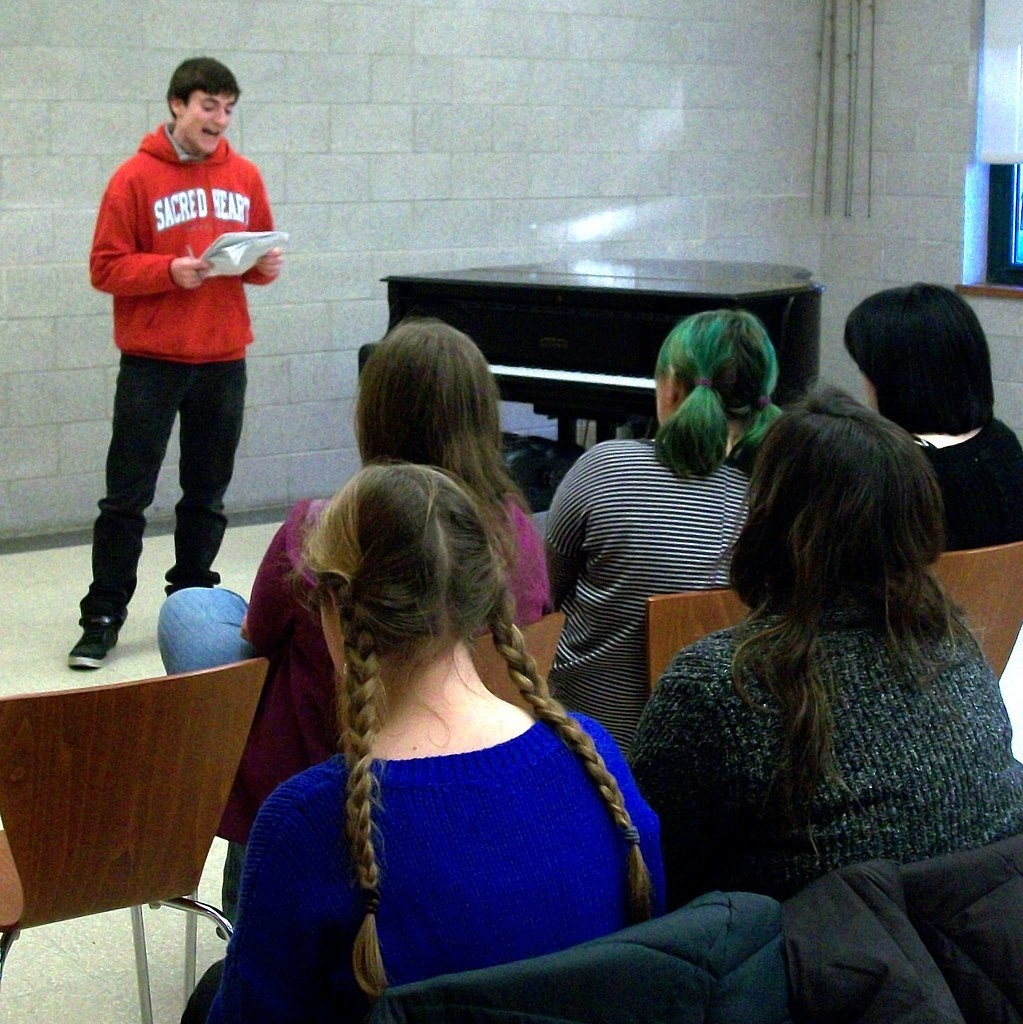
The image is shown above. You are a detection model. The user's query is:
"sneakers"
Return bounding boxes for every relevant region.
[68,620,118,669]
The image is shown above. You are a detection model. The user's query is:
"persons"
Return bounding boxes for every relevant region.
[181,457,671,1024]
[626,378,1022,915]
[543,308,789,765]
[157,315,551,845]
[844,280,1023,552]
[66,55,283,673]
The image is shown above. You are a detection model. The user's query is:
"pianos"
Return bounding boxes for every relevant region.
[359,257,823,445]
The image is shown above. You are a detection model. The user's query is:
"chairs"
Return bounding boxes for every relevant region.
[1,658,279,1023]
[912,537,1021,719]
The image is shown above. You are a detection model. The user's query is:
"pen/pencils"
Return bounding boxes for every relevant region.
[187,243,205,282]
